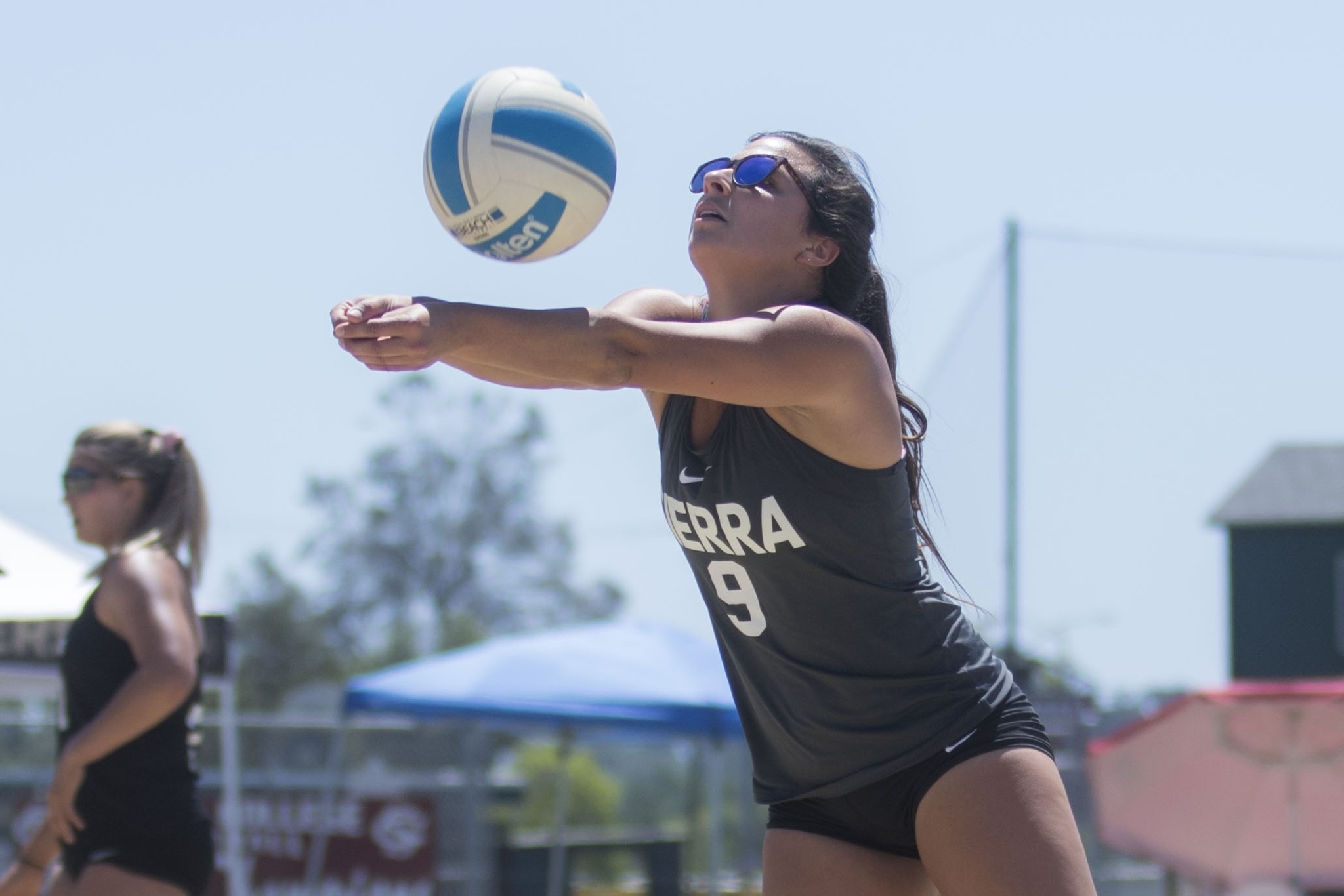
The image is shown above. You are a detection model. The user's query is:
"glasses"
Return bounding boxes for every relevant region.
[688,155,823,222]
[61,468,120,498]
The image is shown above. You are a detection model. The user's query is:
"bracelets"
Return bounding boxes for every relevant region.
[18,859,52,879]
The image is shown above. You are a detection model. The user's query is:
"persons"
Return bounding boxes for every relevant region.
[0,816,59,896]
[46,427,215,895]
[331,127,1096,896]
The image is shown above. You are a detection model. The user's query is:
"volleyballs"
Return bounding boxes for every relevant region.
[423,65,616,263]
